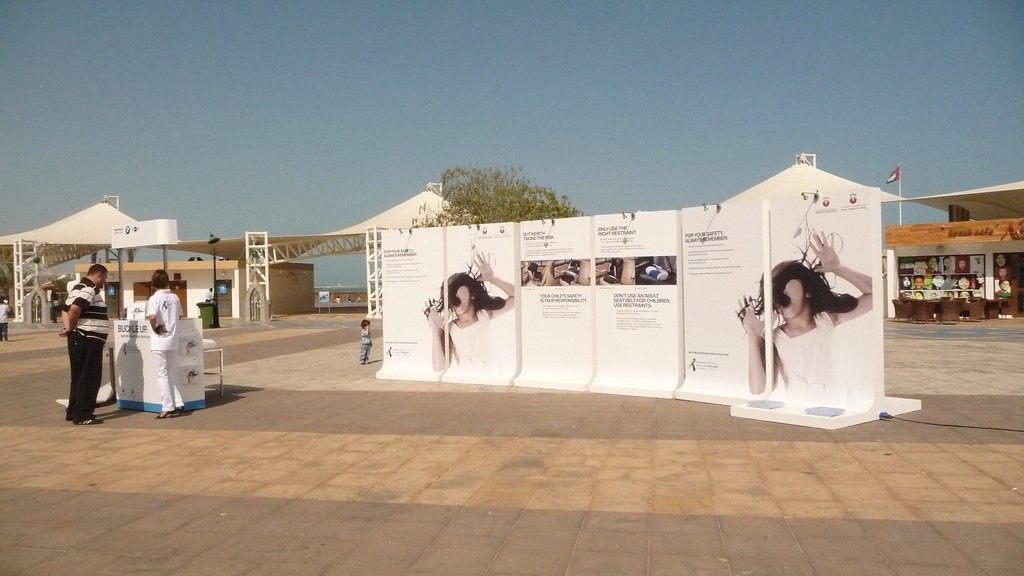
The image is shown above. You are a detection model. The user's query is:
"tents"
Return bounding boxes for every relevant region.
[0,182,486,282]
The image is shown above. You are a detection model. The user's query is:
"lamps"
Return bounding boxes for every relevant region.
[802,192,818,200]
[622,211,635,219]
[541,217,555,226]
[467,223,480,230]
[399,228,412,236]
[703,202,721,211]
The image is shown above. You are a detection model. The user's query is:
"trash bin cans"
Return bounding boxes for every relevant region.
[196,302,215,329]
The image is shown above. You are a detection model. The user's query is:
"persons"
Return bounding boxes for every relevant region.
[333,294,361,304]
[49,294,70,323]
[58,263,110,426]
[145,268,184,419]
[0,296,12,342]
[205,288,214,302]
[734,229,873,406]
[360,320,372,365]
[420,250,516,385]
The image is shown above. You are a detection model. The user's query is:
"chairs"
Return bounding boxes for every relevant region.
[892,295,1012,323]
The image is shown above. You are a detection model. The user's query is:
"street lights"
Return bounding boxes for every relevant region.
[208,231,220,328]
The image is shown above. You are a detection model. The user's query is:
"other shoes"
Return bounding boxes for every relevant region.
[359,361,365,365]
[4,335,8,341]
[365,361,370,364]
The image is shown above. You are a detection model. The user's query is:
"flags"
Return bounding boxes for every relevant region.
[886,166,900,183]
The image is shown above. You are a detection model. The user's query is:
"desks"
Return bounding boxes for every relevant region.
[927,300,941,313]
[988,300,1007,314]
[203,348,224,400]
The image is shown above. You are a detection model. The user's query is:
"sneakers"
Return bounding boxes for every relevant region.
[66,416,73,421]
[74,418,100,425]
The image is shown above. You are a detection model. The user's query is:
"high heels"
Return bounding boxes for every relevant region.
[154,410,174,419]
[175,406,184,416]
